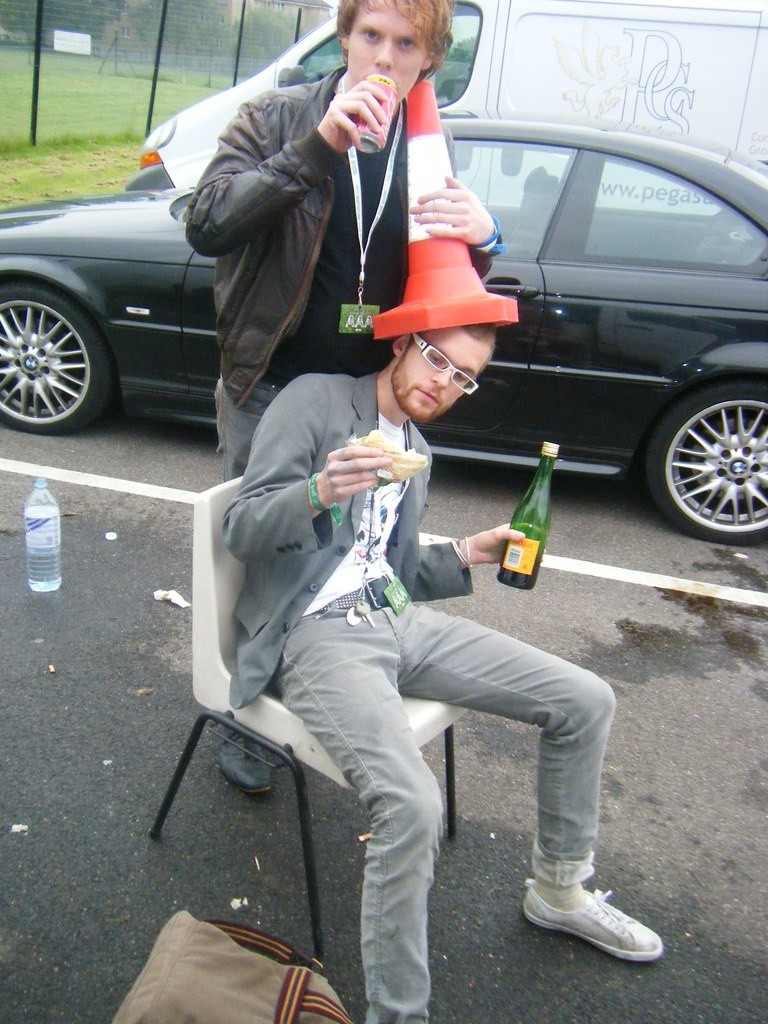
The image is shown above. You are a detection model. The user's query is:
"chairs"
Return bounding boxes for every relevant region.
[150,475,474,962]
[497,166,560,260]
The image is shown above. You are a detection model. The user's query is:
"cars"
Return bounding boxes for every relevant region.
[0,112,768,549]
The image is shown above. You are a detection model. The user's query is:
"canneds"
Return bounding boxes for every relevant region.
[354,74,398,154]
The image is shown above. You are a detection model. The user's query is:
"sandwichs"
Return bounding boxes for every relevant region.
[348,430,428,487]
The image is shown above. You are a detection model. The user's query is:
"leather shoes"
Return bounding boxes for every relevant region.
[218,721,271,792]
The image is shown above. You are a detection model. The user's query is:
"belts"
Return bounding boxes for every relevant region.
[318,573,396,611]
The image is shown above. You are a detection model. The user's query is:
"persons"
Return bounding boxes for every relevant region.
[186,0,505,794]
[222,321,664,1024]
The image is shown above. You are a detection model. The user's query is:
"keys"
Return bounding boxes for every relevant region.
[356,600,375,628]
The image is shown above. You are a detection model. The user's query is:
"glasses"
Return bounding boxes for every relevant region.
[412,332,480,395]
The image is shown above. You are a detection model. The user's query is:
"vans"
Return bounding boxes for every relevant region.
[122,0,768,215]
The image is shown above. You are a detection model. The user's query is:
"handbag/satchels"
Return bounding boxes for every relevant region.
[111,910,356,1024]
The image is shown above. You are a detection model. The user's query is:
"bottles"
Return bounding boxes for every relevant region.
[496,441,562,591]
[24,477,62,593]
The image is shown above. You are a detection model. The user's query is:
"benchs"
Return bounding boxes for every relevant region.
[676,209,768,264]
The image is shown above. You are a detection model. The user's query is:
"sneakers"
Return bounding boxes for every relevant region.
[522,878,664,962]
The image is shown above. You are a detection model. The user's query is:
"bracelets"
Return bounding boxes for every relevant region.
[467,216,508,254]
[309,473,344,526]
[451,537,473,568]
